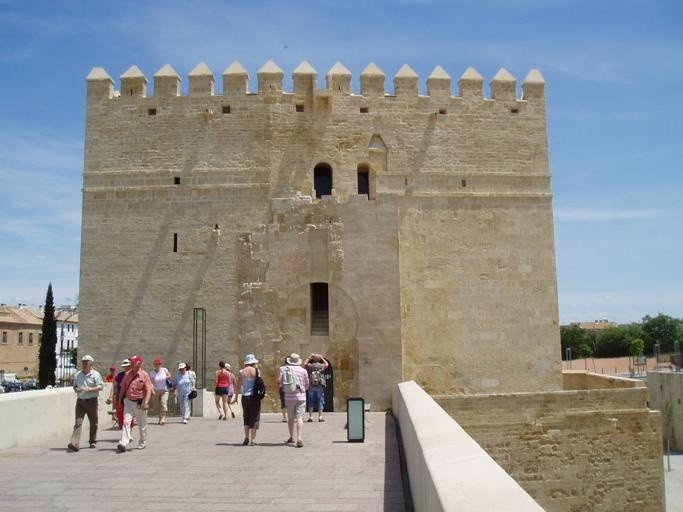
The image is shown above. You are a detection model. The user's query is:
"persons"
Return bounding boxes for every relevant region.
[104,365,117,382]
[67,355,105,451]
[184,363,197,391]
[111,358,137,442]
[212,361,230,420]
[276,352,329,447]
[224,363,236,418]
[116,355,152,452]
[171,362,197,423]
[230,353,261,445]
[148,357,171,425]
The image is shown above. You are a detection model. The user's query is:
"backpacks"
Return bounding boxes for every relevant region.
[312,371,321,386]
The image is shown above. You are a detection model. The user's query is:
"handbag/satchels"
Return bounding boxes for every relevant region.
[120,394,126,405]
[188,390,197,400]
[282,367,297,393]
[253,377,265,400]
[166,379,173,388]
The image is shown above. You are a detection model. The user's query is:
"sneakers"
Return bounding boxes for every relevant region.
[68,444,79,452]
[232,412,235,418]
[297,440,303,447]
[307,419,313,422]
[137,445,143,449]
[90,443,96,448]
[158,421,165,425]
[319,419,324,422]
[183,420,187,424]
[118,444,125,452]
[288,438,295,443]
[251,441,257,445]
[223,418,226,420]
[186,417,191,420]
[219,414,223,420]
[243,438,249,445]
[282,419,286,421]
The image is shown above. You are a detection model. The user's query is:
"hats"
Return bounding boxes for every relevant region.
[153,359,161,365]
[312,354,321,361]
[244,354,259,365]
[225,362,231,371]
[121,359,131,367]
[178,363,187,369]
[82,355,94,362]
[128,355,143,363]
[287,352,302,366]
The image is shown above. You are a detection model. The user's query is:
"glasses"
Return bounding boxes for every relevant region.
[179,368,184,370]
